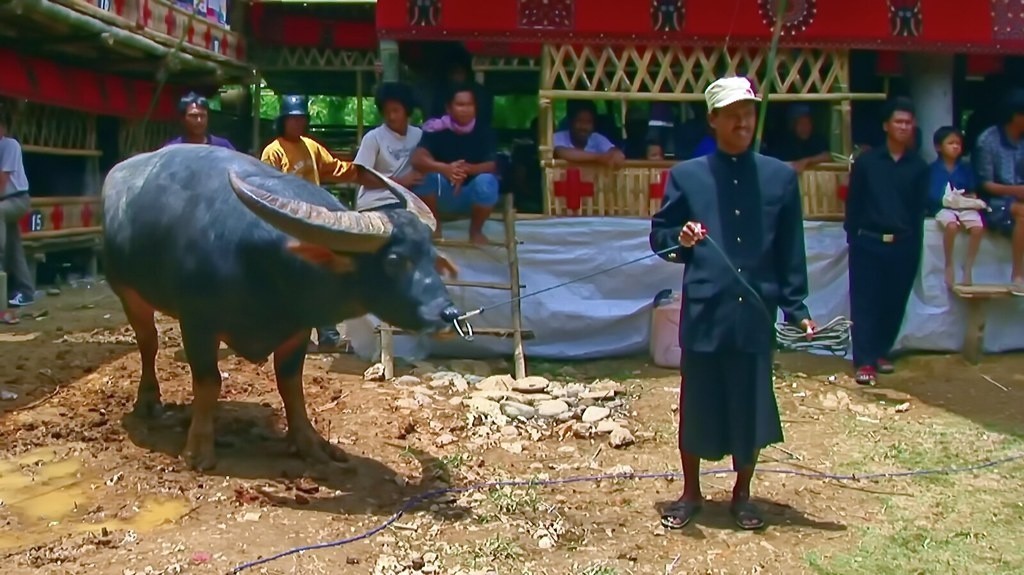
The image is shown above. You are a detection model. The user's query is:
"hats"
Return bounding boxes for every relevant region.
[280,94,308,116]
[705,76,762,115]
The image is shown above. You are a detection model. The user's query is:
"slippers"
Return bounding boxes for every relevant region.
[660,497,705,528]
[729,501,766,530]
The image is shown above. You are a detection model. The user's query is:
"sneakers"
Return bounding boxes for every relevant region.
[8,290,36,306]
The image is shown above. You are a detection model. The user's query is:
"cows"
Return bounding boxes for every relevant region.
[96,143,464,472]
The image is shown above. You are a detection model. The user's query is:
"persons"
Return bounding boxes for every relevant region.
[0,106,35,324]
[410,88,497,245]
[354,81,435,213]
[929,126,986,292]
[150,91,237,152]
[259,95,357,353]
[508,102,833,213]
[650,75,815,529]
[972,103,1024,297]
[843,96,930,383]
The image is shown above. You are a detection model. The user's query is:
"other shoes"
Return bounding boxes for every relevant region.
[875,358,898,373]
[856,364,877,384]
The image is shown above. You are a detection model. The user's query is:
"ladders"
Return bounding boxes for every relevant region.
[374,193,535,380]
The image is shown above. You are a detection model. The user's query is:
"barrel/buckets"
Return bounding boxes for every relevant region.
[649,288,681,370]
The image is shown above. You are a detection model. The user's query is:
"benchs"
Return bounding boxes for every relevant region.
[951,284,1014,365]
